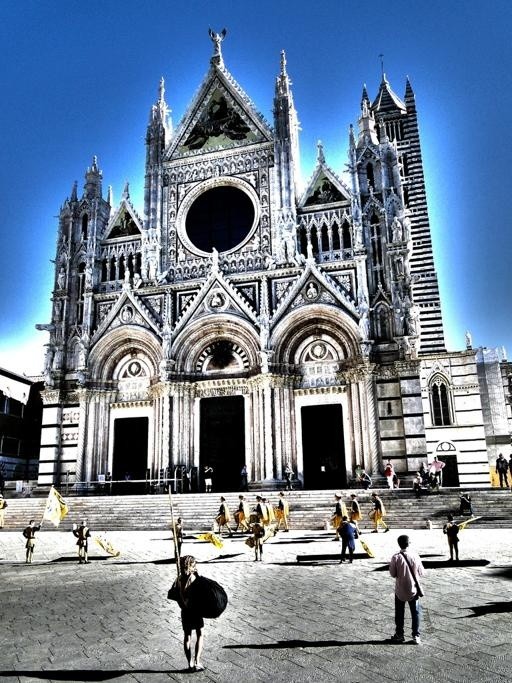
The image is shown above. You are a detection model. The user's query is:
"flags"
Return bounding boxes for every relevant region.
[43,487,68,528]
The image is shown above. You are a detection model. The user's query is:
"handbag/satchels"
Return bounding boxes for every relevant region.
[167,581,181,600]
[185,570,228,619]
[415,582,424,597]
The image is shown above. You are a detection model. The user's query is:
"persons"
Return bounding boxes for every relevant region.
[389,534,426,645]
[168,555,207,671]
[459,491,475,518]
[172,457,446,564]
[124,472,132,495]
[104,472,111,496]
[72,521,92,564]
[23,519,43,564]
[509,454,512,479]
[443,514,459,561]
[495,453,510,487]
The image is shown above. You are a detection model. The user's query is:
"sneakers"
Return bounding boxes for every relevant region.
[391,633,405,642]
[189,664,207,672]
[413,635,420,644]
[0,484,510,563]
